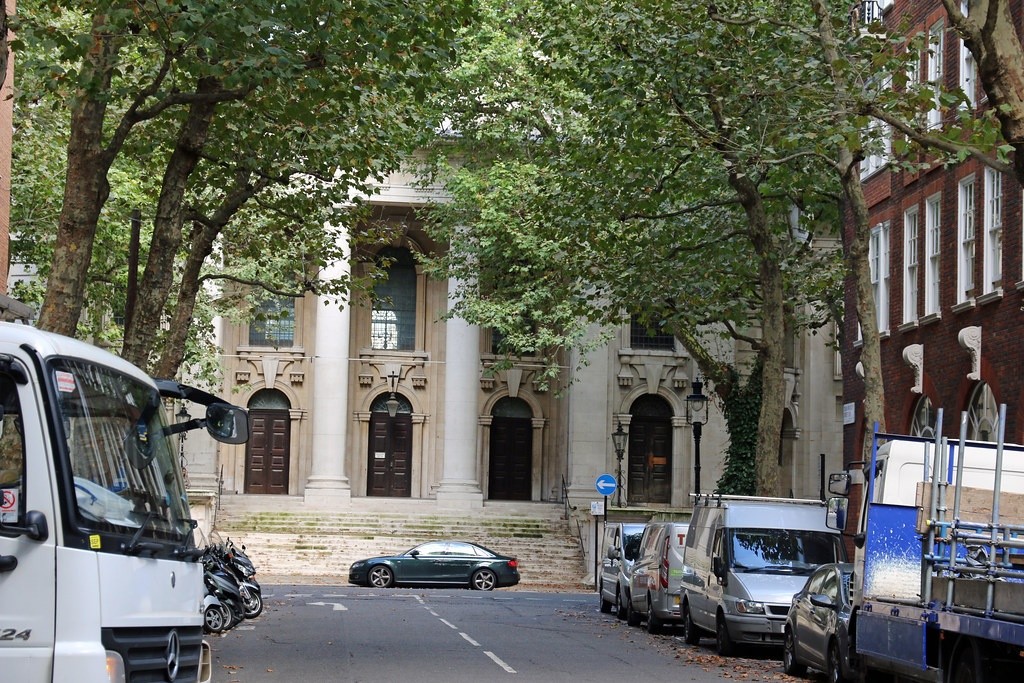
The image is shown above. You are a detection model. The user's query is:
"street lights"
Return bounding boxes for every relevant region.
[610,419,629,509]
[683,376,710,506]
[385,393,400,497]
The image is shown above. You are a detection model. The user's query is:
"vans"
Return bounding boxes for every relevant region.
[680,493,849,656]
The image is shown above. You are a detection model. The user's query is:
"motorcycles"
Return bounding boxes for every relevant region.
[198,533,263,634]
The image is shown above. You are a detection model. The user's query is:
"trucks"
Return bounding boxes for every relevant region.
[825,403,1024,683]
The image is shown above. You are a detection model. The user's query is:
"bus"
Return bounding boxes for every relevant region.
[0,322,253,683]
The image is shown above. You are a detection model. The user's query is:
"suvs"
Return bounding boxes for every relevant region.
[599,522,648,620]
[627,522,691,629]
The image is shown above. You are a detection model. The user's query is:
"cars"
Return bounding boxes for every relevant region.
[348,540,520,592]
[784,562,854,683]
